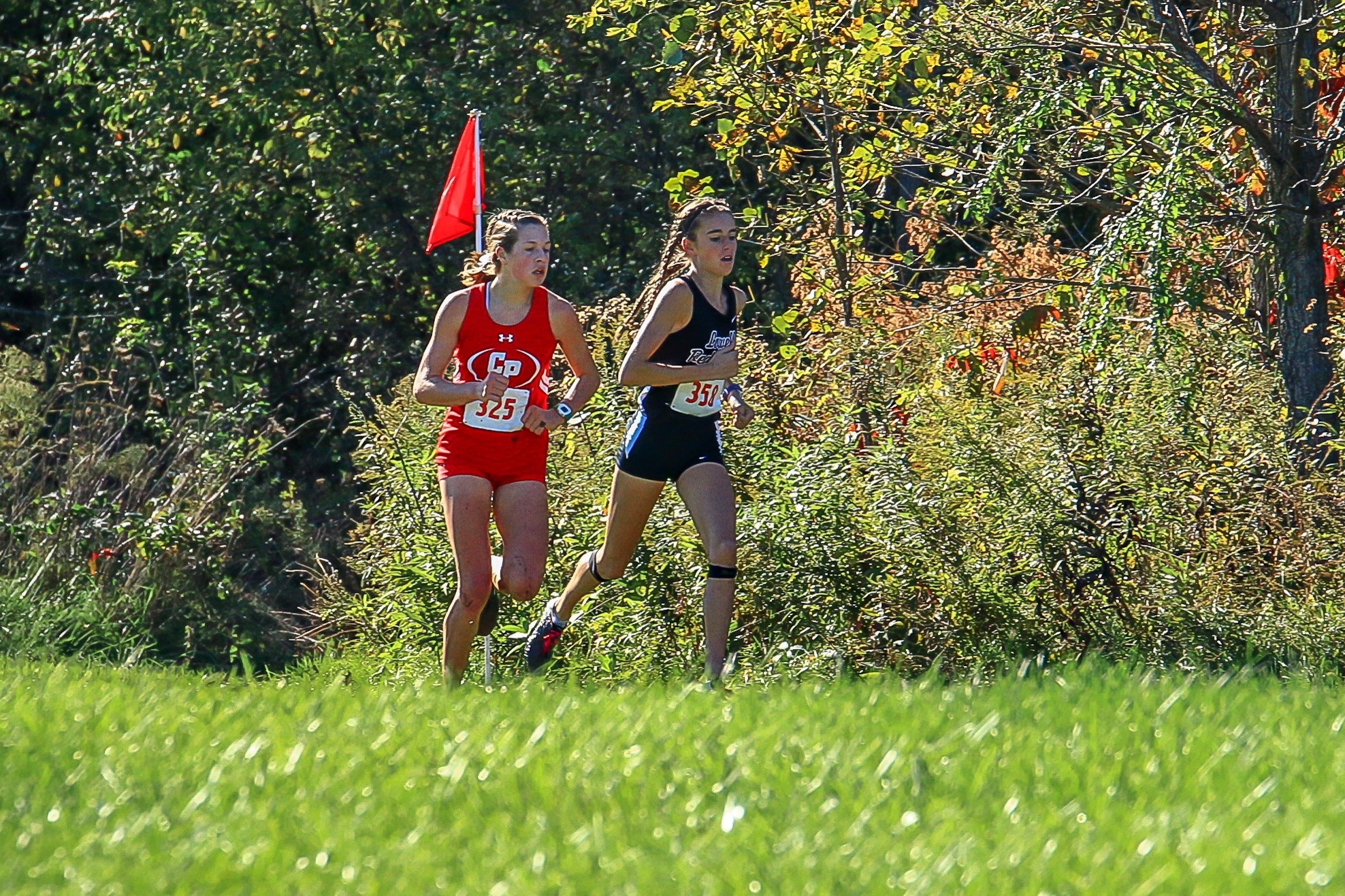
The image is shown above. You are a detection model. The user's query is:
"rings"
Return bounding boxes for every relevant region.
[538,421,546,428]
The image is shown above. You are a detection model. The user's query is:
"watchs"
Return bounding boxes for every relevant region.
[553,402,572,421]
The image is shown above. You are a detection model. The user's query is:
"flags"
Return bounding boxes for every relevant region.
[423,115,485,253]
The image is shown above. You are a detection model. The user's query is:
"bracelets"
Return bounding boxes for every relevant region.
[722,384,743,402]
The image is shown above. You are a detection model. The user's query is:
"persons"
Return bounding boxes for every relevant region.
[415,207,601,690]
[523,197,755,688]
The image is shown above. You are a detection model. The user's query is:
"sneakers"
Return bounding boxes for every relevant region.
[522,597,566,673]
[478,583,499,636]
